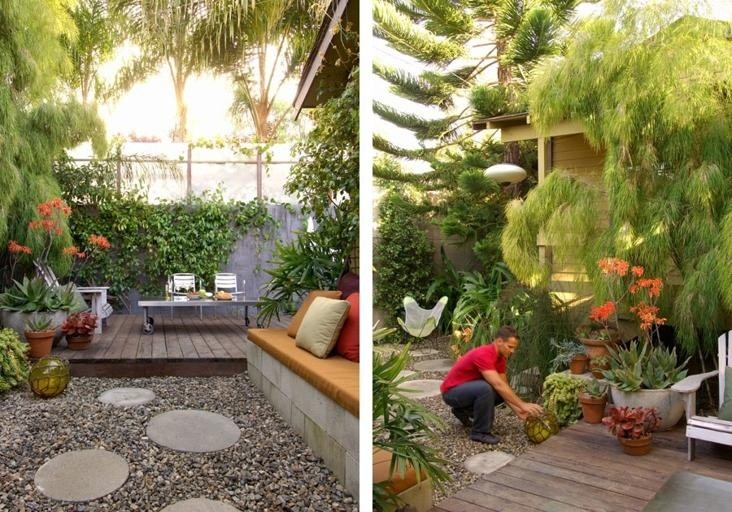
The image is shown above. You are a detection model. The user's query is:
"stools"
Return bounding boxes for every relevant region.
[372,445,434,512]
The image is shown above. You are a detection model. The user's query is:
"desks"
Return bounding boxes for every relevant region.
[137,298,267,335]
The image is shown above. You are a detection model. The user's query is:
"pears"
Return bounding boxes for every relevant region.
[198,289,206,298]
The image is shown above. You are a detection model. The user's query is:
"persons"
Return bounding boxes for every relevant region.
[439,325,543,444]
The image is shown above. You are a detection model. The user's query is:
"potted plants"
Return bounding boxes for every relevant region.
[551,322,661,457]
[61,312,100,350]
[23,313,56,358]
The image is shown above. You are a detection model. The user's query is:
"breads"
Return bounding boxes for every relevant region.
[216,290,233,299]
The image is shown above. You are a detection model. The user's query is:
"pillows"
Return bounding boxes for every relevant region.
[286,289,341,340]
[295,296,352,357]
[331,292,360,362]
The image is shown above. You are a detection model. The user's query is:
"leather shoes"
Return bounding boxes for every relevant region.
[451,407,473,427]
[469,431,500,444]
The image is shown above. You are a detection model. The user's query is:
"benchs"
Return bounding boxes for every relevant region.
[245,327,359,504]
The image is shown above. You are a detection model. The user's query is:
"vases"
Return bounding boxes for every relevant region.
[606,379,688,433]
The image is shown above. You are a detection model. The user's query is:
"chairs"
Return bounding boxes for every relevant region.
[166,272,204,319]
[667,329,732,462]
[31,258,113,328]
[213,271,246,320]
[396,295,449,354]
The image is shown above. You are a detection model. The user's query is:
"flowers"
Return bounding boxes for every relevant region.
[585,256,693,393]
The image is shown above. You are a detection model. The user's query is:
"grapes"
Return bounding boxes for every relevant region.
[186,290,198,301]
